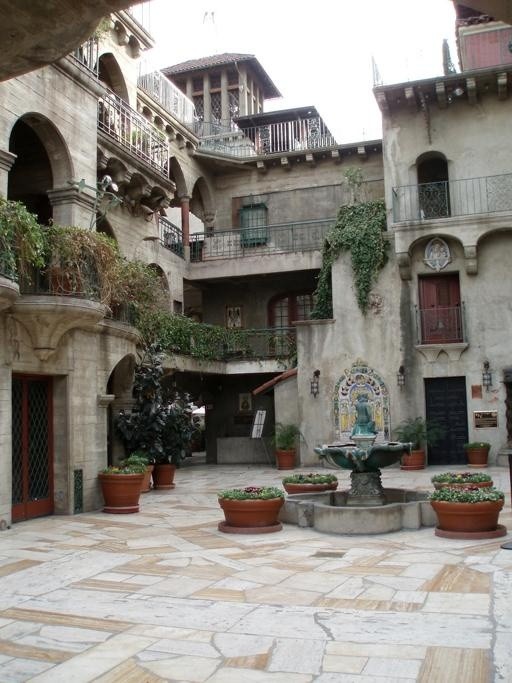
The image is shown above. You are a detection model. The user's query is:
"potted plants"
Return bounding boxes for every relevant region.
[260,421,305,469]
[427,471,505,532]
[98,345,202,507]
[216,472,338,526]
[0,194,168,322]
[391,415,445,469]
[463,441,492,463]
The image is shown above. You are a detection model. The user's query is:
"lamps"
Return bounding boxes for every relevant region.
[482,360,492,391]
[397,365,406,390]
[311,369,320,397]
